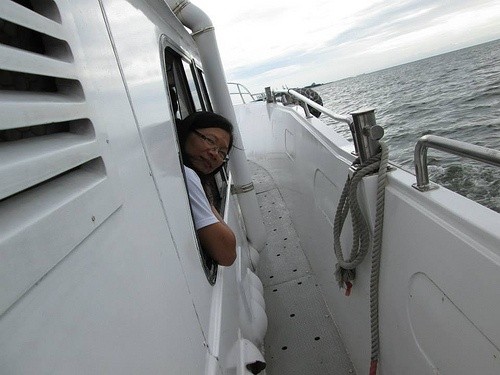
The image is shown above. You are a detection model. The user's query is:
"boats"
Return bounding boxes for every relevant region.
[1,0,500,374]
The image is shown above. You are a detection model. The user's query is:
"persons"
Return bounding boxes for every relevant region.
[176,112,238,267]
